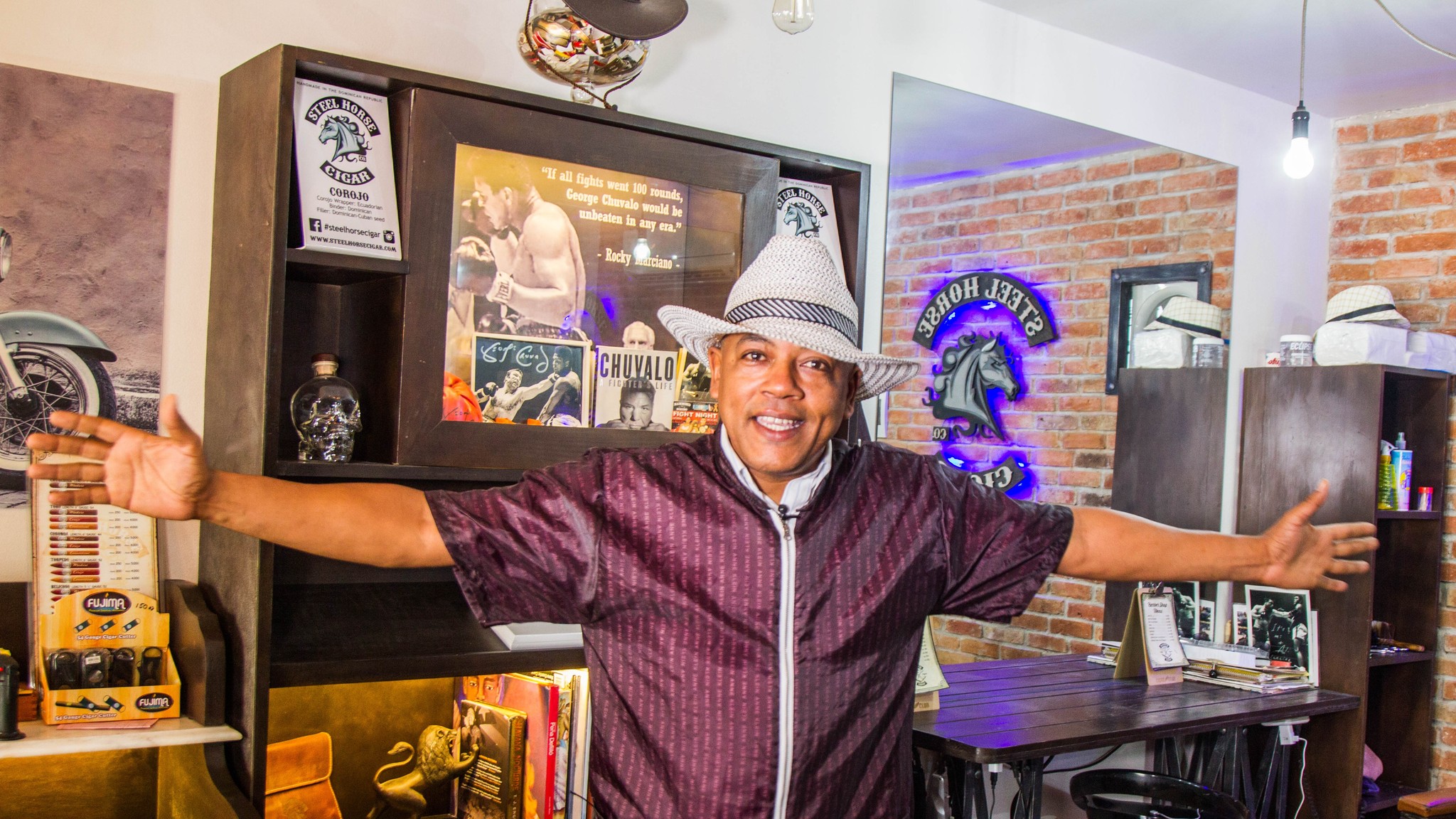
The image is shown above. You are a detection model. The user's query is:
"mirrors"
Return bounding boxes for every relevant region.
[879,72,1242,664]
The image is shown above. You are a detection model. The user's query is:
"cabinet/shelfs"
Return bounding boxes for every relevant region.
[198,43,871,819]
[1102,368,1228,653]
[1103,364,1452,819]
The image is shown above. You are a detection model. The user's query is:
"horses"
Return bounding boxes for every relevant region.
[920,331,1021,441]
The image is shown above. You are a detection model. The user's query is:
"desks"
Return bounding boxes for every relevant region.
[939,652,1118,679]
[911,679,1360,819]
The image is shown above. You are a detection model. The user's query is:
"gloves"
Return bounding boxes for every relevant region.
[461,192,498,236]
[548,368,559,383]
[449,236,497,297]
[482,382,499,397]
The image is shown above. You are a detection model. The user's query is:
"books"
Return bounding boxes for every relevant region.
[450,669,590,819]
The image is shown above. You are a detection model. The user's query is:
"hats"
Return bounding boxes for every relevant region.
[657,235,921,403]
[1311,285,1411,344]
[1144,294,1224,344]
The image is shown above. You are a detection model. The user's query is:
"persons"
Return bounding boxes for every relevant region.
[477,369,560,423]
[1251,594,1308,667]
[1172,587,1195,638]
[623,321,655,350]
[462,707,483,746]
[26,234,1379,819]
[596,377,670,431]
[538,346,581,426]
[681,360,706,401]
[674,416,710,434]
[454,148,587,338]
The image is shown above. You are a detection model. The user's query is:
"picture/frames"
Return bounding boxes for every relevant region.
[1232,584,1320,689]
[1104,260,1212,395]
[1138,579,1215,642]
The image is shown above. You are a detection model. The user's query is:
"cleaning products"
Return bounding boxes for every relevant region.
[1378,432,1414,512]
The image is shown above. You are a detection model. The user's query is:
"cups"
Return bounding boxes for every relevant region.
[1191,337,1223,367]
[1266,334,1312,367]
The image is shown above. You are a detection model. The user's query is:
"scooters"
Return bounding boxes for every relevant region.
[1,225,121,497]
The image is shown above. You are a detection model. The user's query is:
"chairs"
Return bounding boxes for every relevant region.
[1070,768,1250,819]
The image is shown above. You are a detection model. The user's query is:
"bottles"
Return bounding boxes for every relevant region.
[289,354,361,463]
[1417,487,1433,511]
[0,654,20,738]
[632,237,651,260]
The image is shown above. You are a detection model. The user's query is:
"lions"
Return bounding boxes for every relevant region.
[367,724,479,819]
